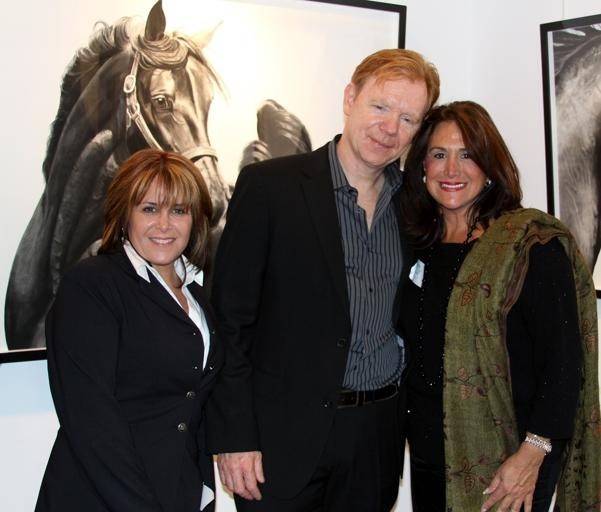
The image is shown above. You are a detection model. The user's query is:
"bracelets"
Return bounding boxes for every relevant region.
[523,434,554,456]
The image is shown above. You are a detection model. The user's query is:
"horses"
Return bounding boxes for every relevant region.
[4,0,313,351]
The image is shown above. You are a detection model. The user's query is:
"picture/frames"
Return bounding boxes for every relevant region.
[0,0,408,364]
[540,13,601,302]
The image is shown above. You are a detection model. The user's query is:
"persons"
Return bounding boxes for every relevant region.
[389,98,601,512]
[205,47,441,511]
[33,148,229,512]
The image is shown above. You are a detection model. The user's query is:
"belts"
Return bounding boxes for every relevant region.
[335,380,400,408]
